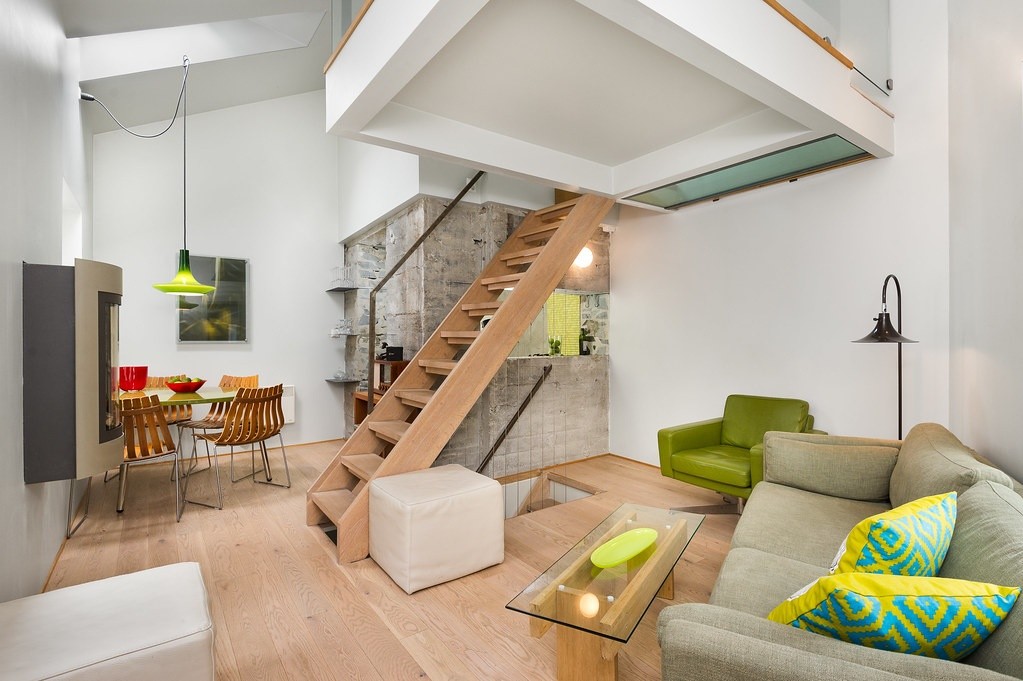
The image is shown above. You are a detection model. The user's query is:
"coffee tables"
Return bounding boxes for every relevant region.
[505,502,705,680]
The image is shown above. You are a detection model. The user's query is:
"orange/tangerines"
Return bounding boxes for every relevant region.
[168,377,202,383]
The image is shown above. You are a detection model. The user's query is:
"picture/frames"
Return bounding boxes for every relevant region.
[176,252,249,345]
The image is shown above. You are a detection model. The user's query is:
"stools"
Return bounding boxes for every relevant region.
[367,462,505,596]
[0,562,218,681]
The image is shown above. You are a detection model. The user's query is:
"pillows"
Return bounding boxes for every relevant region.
[830,491,958,575]
[770,572,1023,662]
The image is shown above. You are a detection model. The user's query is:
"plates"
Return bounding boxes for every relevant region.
[590,527,658,568]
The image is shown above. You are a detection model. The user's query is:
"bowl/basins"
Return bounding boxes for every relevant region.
[119,365,148,392]
[165,380,206,393]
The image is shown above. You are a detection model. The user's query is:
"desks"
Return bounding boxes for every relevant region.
[116,386,273,513]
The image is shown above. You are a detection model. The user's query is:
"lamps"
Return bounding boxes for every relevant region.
[850,274,919,440]
[78,54,216,298]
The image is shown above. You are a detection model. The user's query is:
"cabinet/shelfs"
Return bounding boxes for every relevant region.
[326,286,359,385]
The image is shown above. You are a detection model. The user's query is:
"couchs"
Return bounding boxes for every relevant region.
[656,422,1023,681]
[658,394,827,515]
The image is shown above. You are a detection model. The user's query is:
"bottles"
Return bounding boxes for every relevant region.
[579,328,587,355]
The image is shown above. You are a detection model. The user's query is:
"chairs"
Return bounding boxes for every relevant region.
[170,375,265,482]
[181,382,292,510]
[68,395,186,539]
[102,376,198,483]
